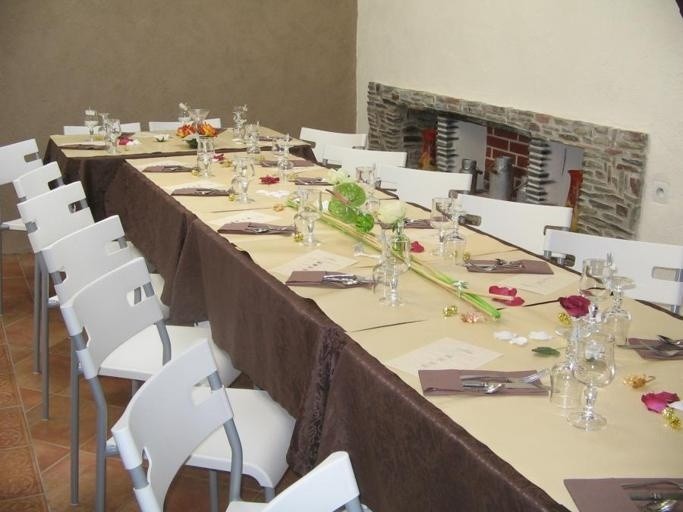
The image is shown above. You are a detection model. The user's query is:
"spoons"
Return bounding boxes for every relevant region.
[656,334,683,344]
[640,499,678,512]
[421,382,505,395]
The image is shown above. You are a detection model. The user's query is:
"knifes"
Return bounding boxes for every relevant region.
[628,493,682,500]
[617,343,683,349]
[464,381,560,394]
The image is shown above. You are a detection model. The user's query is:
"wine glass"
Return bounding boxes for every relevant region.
[84,108,121,154]
[550,258,635,429]
[228,155,256,204]
[373,217,411,307]
[177,104,211,125]
[298,188,325,265]
[269,132,293,190]
[356,166,380,216]
[431,197,468,264]
[230,106,262,155]
[196,135,216,178]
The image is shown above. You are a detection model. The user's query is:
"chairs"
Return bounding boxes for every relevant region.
[300,127,366,166]
[340,148,407,179]
[40,215,242,506]
[542,226,683,316]
[110,339,243,512]
[224,451,372,512]
[61,257,296,503]
[0,138,47,232]
[13,162,65,202]
[17,182,164,376]
[63,118,221,136]
[453,193,572,256]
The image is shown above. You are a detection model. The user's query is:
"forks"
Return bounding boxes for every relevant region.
[639,340,683,357]
[459,369,547,381]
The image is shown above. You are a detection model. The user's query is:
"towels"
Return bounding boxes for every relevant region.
[628,338,683,360]
[564,478,683,512]
[57,144,107,150]
